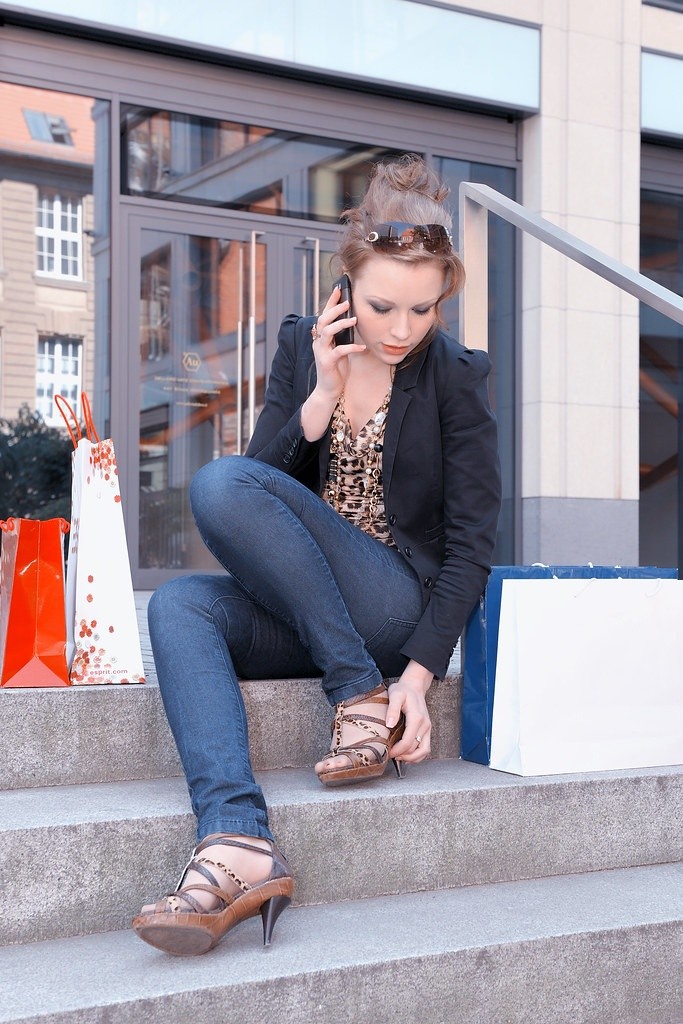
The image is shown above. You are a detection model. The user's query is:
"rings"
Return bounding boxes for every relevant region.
[416,736,423,742]
[310,325,321,340]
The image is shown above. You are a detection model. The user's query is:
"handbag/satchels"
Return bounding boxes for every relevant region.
[53,392,148,688]
[0,514,73,689]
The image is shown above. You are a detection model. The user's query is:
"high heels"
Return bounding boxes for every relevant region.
[131,830,296,956]
[316,683,405,787]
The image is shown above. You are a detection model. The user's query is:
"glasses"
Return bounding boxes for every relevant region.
[360,221,454,256]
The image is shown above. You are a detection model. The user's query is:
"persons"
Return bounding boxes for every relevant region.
[134,157,501,957]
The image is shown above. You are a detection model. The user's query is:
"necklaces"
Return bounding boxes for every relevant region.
[328,380,393,532]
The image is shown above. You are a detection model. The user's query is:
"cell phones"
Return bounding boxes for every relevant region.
[331,273,355,347]
